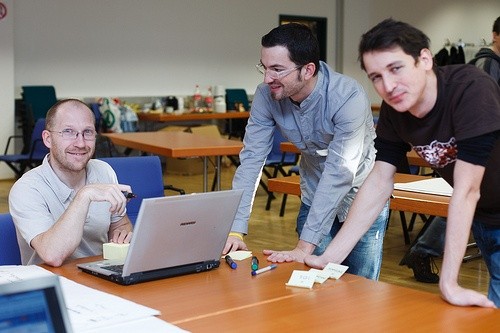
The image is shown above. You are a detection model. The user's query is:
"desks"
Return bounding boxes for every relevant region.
[101,132,243,192]
[281,141,435,167]
[267,172,451,216]
[136,111,249,125]
[35,253,500,333]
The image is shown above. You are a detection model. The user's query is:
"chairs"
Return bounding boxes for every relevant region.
[0,85,482,333]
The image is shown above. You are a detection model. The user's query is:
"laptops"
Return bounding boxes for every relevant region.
[76,188,245,285]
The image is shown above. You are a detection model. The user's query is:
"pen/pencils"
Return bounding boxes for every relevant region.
[251,264,278,275]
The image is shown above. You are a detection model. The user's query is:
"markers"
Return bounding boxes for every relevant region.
[122,191,134,197]
[226,254,236,268]
[251,256,260,271]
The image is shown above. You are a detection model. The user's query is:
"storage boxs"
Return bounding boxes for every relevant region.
[103,242,130,259]
[160,126,221,176]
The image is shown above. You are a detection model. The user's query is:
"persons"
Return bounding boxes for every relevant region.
[221,23,389,282]
[9,99,132,267]
[302,18,500,310]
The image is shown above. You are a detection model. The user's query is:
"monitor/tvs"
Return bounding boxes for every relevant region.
[0,265,71,333]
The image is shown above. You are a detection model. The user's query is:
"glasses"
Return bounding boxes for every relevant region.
[47,127,98,141]
[255,63,303,80]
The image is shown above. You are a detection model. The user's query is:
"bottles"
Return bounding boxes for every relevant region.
[205,87,214,113]
[193,85,202,114]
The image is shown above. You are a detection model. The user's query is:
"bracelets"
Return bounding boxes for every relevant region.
[229,233,243,241]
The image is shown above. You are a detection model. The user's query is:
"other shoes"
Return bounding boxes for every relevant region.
[405,245,440,284]
